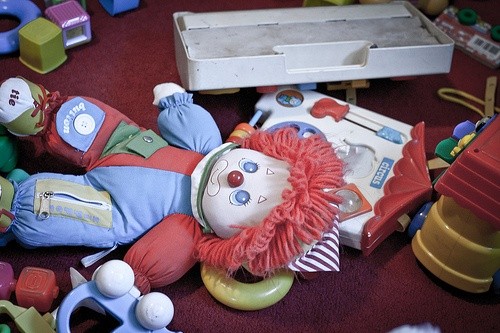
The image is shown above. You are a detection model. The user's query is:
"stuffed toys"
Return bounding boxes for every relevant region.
[0,75,348,298]
